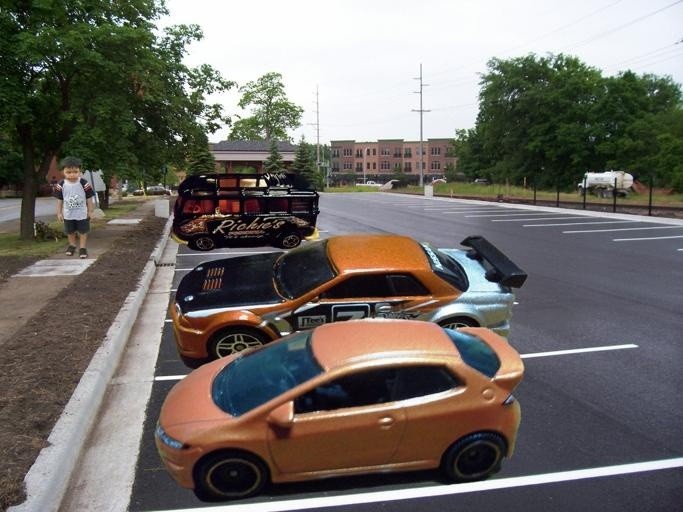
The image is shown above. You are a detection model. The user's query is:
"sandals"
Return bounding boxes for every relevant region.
[80,248,87,258]
[66,246,76,255]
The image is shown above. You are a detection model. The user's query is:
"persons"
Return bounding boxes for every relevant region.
[48,174,57,192]
[54,156,95,259]
